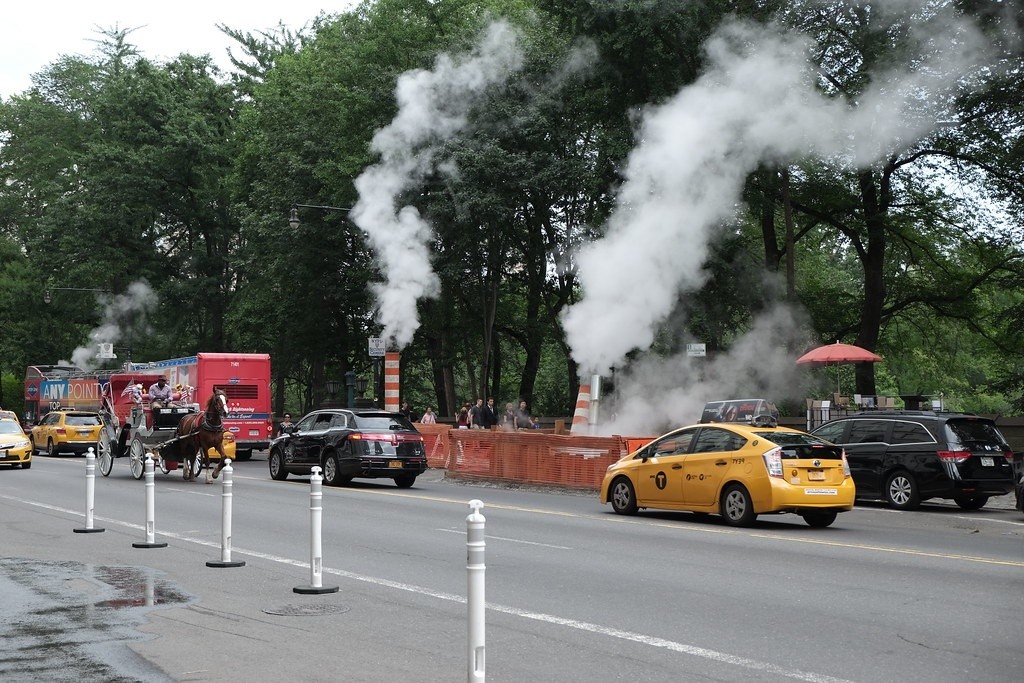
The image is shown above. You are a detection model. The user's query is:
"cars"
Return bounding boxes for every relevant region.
[26,404,105,457]
[0,406,33,469]
[598,398,856,528]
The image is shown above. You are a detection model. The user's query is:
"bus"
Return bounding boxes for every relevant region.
[23,352,272,460]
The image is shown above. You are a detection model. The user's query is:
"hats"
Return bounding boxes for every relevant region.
[157,375,167,381]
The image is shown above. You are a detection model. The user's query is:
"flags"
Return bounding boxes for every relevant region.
[179,389,187,400]
[120,379,133,397]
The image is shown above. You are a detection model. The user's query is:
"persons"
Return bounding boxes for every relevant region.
[421,407,436,424]
[531,416,542,429]
[514,401,533,431]
[724,404,737,422]
[277,413,294,438]
[399,400,411,421]
[149,375,177,411]
[455,397,499,429]
[504,403,514,423]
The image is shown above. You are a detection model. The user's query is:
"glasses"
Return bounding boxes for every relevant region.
[285,416,290,418]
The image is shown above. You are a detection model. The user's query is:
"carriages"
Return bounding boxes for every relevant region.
[96,368,231,485]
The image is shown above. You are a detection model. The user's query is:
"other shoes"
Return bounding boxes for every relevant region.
[153,423,159,431]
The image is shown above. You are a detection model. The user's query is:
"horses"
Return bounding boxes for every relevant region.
[175,386,230,485]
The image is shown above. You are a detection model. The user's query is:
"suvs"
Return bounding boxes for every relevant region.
[803,406,1016,511]
[267,406,429,490]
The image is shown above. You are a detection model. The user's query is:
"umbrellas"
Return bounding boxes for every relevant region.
[796,340,884,394]
